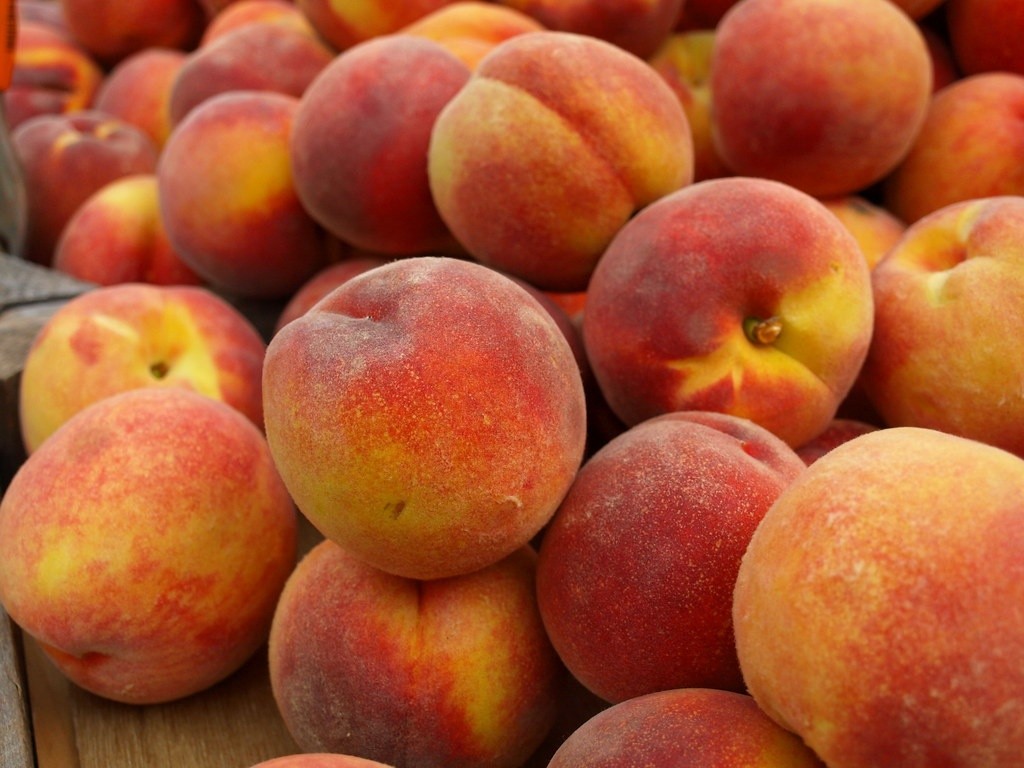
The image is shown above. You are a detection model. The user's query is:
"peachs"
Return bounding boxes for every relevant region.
[0,0,1024,768]
